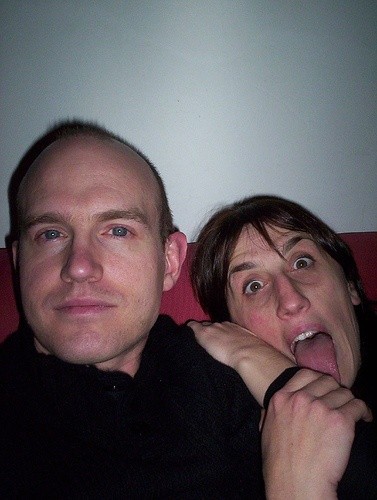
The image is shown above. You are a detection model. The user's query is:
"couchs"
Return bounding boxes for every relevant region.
[0,231,377,347]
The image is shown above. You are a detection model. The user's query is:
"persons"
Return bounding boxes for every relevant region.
[189,197,377,500]
[0,126,267,499]
